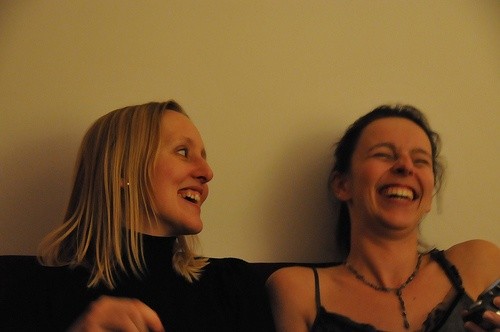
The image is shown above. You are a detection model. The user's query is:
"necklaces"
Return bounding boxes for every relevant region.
[341,250,423,330]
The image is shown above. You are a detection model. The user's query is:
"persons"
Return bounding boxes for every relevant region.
[0,98,280,332]
[265,102,499,332]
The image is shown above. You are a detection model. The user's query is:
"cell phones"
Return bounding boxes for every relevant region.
[467,280,500,323]
